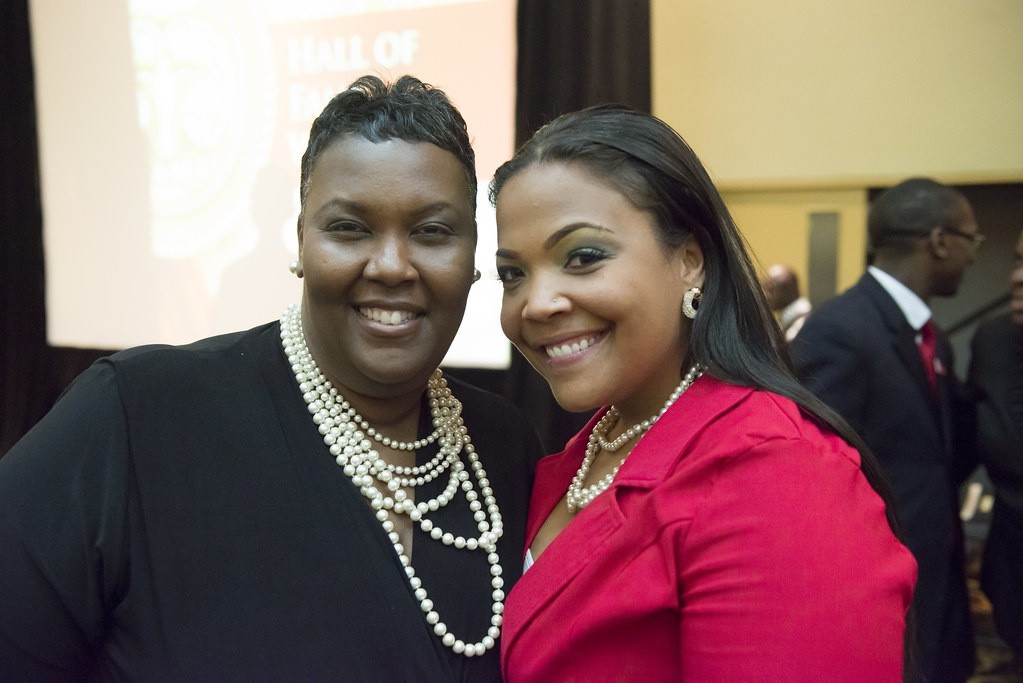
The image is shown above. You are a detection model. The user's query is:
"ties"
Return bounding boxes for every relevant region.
[918,319,940,392]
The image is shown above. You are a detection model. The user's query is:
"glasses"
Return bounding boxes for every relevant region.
[922,227,984,249]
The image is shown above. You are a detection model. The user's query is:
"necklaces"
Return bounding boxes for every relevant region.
[278,299,505,657]
[565,360,709,516]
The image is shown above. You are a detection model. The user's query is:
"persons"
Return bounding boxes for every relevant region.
[787,179,986,683]
[966,246,1023,672]
[763,264,812,343]
[488,104,919,683]
[0,75,536,683]
